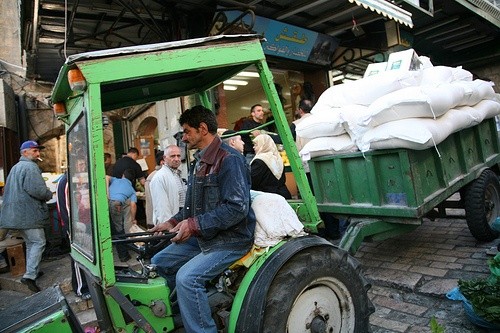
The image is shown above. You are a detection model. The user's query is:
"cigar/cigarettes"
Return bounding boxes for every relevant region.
[37,157,43,162]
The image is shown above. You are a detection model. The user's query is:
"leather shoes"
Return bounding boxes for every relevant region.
[20,278,41,292]
[36,272,43,277]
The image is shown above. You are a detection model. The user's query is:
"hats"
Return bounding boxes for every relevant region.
[20,141,45,149]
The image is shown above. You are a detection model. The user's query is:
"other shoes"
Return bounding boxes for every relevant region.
[120,255,131,263]
[81,291,91,300]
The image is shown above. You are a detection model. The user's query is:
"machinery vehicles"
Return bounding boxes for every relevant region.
[0,33,500,333]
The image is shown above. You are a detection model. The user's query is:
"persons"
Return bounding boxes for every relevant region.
[149,145,186,230]
[56,148,165,301]
[148,105,256,333]
[221,104,277,162]
[2,141,53,294]
[250,135,292,199]
[270,100,314,199]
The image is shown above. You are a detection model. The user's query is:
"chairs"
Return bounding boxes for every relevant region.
[217,190,304,292]
